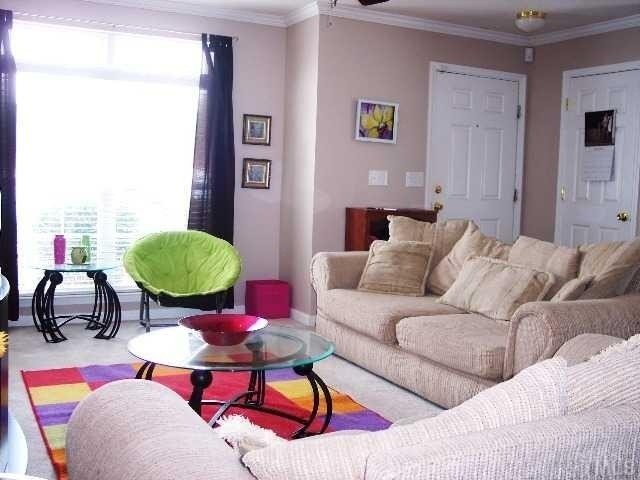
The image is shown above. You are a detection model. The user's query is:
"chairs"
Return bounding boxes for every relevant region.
[122,229,244,334]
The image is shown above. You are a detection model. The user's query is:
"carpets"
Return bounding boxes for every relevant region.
[20,352,398,478]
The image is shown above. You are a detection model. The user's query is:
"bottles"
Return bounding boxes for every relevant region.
[69,246,88,266]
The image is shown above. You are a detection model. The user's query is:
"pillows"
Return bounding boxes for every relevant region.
[559,330,640,406]
[239,354,571,479]
[356,215,639,323]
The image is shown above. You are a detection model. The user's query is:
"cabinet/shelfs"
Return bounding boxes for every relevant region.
[345,207,439,252]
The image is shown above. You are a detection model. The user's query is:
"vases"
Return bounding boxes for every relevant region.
[70,245,88,263]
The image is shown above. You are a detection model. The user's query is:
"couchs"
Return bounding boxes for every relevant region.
[308,250,640,412]
[64,329,639,478]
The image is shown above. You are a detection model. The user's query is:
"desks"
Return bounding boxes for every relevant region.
[30,260,122,344]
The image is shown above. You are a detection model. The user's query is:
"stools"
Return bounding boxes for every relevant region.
[244,278,292,320]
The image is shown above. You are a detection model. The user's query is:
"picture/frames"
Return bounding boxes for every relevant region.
[240,158,271,189]
[241,114,271,146]
[353,98,400,146]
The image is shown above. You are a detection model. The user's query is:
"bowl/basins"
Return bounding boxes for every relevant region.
[176,313,271,348]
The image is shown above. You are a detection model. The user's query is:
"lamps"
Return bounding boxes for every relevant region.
[515,7,549,34]
